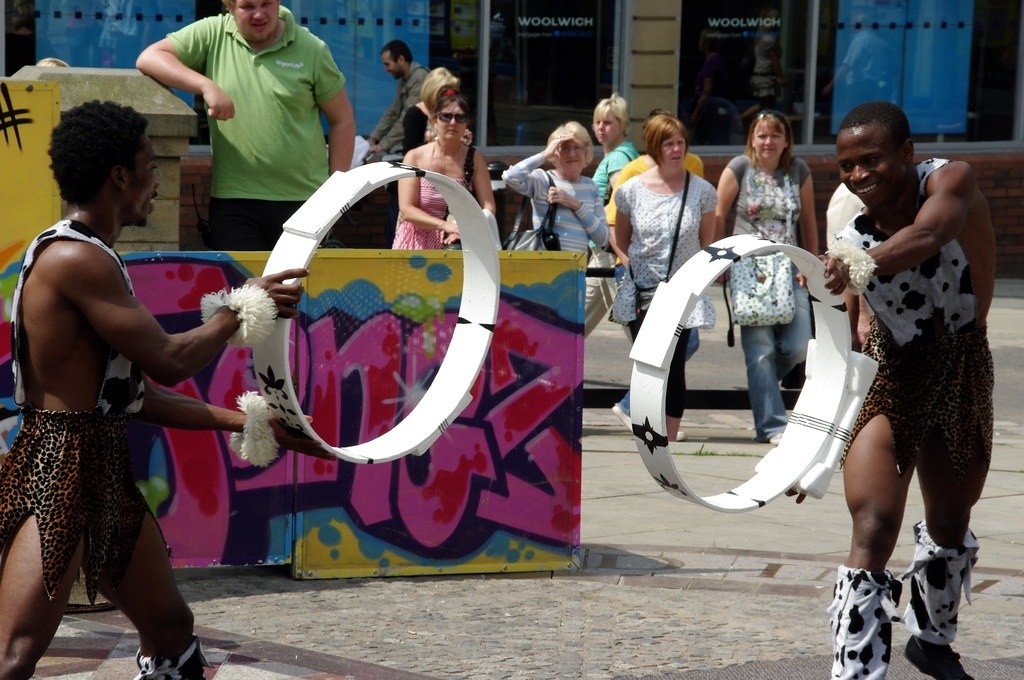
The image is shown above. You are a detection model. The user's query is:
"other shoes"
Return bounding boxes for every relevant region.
[768,432,784,445]
[675,430,688,441]
[611,403,634,433]
[903,635,975,680]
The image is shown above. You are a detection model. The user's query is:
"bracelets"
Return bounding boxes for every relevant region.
[825,237,878,295]
[228,391,279,467]
[200,284,278,345]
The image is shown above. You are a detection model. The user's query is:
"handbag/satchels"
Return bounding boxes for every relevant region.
[730,252,796,327]
[635,278,671,316]
[503,170,562,250]
[442,146,476,244]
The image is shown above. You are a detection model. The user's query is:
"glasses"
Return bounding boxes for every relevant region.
[556,143,586,154]
[756,108,786,130]
[435,111,470,124]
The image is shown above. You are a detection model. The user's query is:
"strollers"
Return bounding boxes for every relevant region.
[320,135,388,248]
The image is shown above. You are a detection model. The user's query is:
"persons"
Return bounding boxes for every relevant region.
[501,121,610,261]
[795,100,997,680]
[0,101,310,680]
[136,0,354,252]
[582,94,818,445]
[365,39,496,250]
